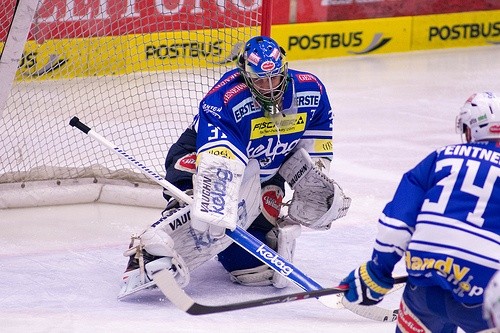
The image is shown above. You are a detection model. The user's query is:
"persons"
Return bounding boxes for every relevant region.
[337,92,500,333]
[117,37,351,299]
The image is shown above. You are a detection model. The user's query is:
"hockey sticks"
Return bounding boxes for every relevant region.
[69,116,404,310]
[152,269,410,315]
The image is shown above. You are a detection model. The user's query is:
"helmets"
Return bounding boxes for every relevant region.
[459,91,500,144]
[237,35,288,114]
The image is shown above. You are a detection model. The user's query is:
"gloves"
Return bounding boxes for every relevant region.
[338,260,394,307]
